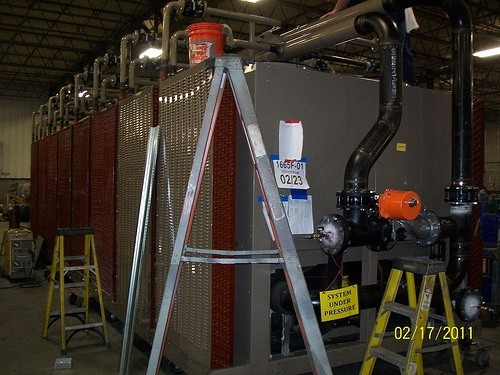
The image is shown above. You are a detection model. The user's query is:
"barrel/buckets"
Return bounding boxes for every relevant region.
[185,21,224,69]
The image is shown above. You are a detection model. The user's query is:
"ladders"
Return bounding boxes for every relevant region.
[141,54,338,375]
[43,226,112,354]
[357,254,466,375]
